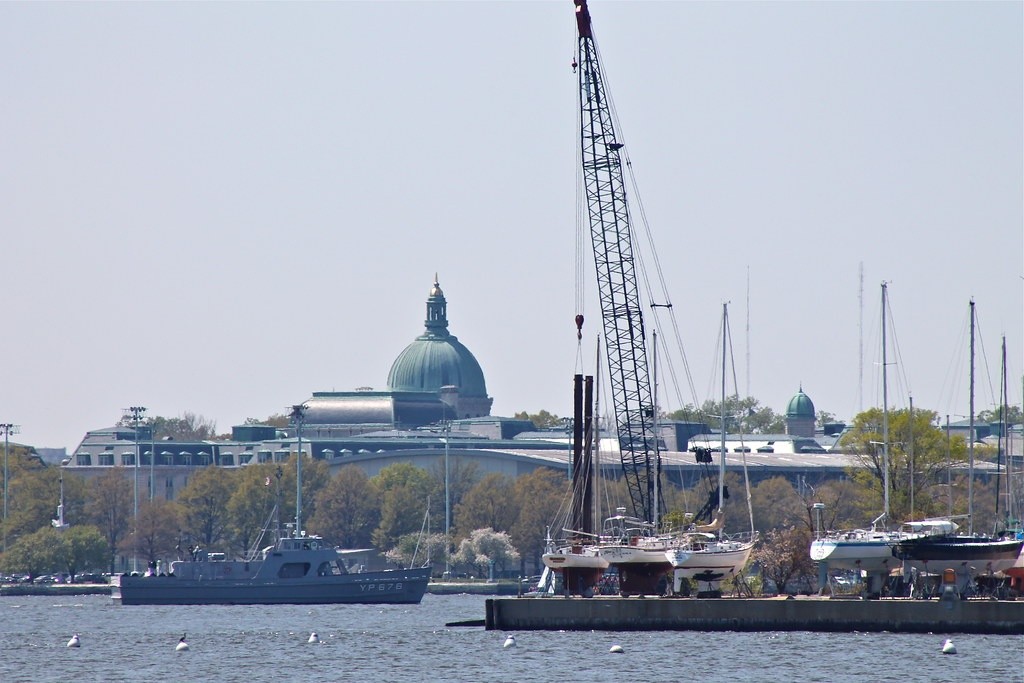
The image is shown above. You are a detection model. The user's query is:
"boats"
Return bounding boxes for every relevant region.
[105,466,433,604]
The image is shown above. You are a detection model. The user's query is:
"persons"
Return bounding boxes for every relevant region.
[517,576,523,598]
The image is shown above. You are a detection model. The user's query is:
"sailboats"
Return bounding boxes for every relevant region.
[807,282,1024,594]
[538,299,760,582]
